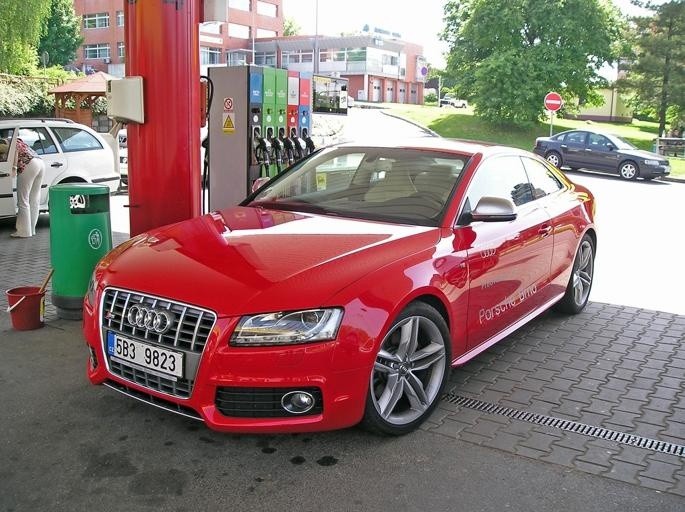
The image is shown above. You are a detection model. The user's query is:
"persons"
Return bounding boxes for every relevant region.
[0,137,45,238]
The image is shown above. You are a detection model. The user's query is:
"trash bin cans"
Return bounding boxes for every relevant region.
[50,184,111,310]
[653,139,656,152]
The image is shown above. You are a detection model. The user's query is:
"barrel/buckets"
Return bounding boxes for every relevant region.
[5,286,46,330]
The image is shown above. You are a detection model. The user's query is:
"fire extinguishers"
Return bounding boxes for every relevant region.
[199,77,213,127]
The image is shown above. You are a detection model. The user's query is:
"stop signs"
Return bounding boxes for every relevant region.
[544,92,563,112]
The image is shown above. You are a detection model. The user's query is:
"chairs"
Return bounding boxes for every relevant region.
[365,162,417,202]
[414,165,458,198]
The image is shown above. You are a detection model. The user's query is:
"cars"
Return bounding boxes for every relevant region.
[83,139,598,436]
[532,128,671,181]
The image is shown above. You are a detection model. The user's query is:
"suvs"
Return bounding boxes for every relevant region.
[0,119,122,222]
[439,93,468,109]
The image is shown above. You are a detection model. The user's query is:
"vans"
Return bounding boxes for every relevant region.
[315,90,348,114]
